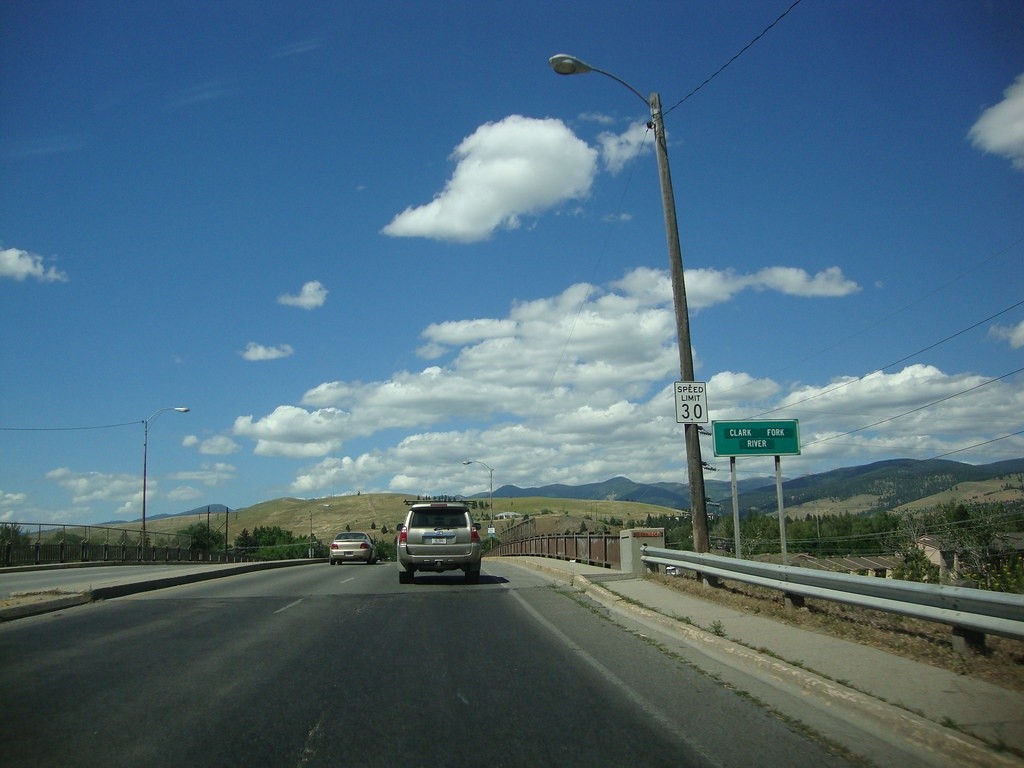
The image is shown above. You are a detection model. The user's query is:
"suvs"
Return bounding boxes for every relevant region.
[395,498,484,585]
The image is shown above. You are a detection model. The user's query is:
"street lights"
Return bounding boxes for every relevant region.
[140,407,191,562]
[548,52,713,583]
[309,504,330,558]
[462,461,494,551]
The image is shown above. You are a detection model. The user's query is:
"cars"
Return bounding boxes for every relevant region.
[328,531,378,565]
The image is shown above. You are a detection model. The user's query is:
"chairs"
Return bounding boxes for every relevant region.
[434,520,446,526]
[449,518,461,525]
[417,517,429,526]
